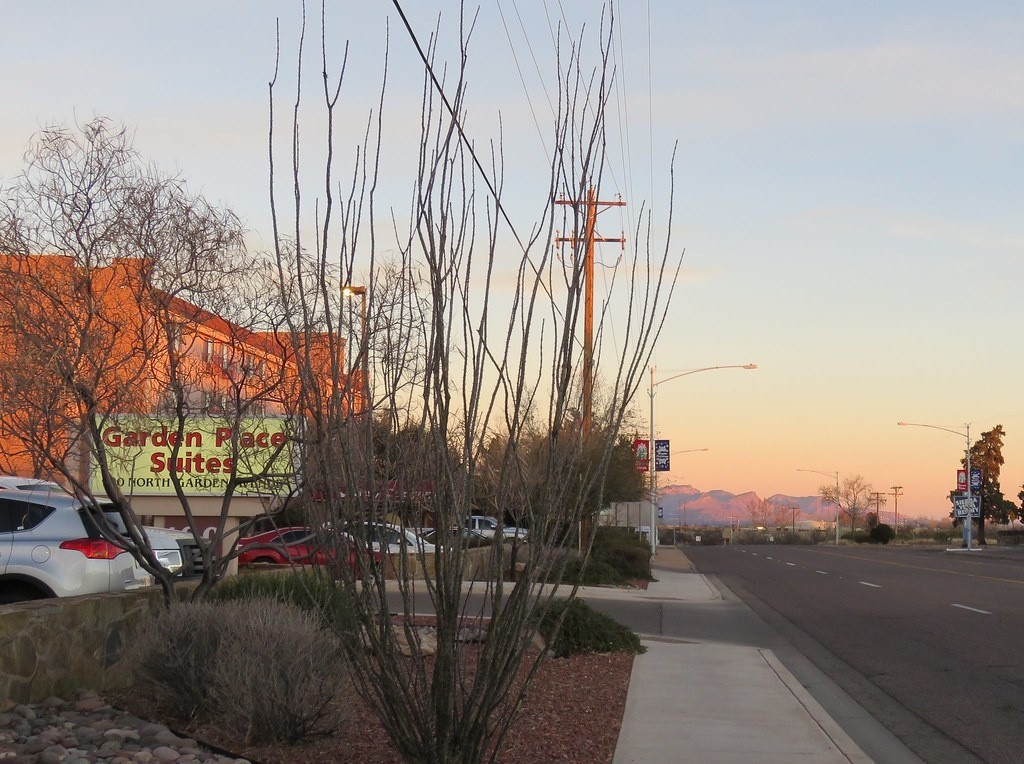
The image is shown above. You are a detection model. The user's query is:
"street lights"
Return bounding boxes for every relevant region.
[650,364,758,558]
[797,469,839,545]
[342,286,367,439]
[897,422,971,550]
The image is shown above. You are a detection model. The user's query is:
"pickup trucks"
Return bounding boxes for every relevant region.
[407,514,529,545]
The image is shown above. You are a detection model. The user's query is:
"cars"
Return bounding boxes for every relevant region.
[417,528,493,550]
[239,527,383,580]
[322,521,453,553]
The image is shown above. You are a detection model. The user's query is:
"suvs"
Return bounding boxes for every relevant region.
[0,477,216,606]
